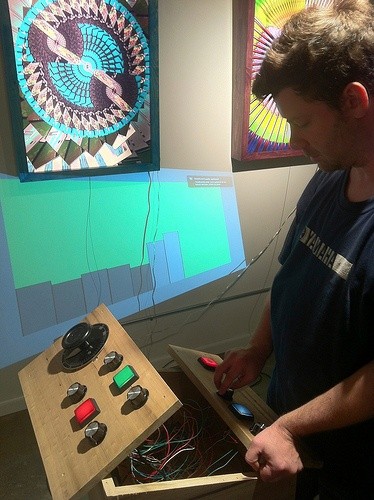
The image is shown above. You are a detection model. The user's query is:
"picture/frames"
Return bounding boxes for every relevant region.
[230,0,338,164]
[0,0,163,184]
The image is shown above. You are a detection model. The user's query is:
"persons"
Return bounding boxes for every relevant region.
[214,0,374,500]
